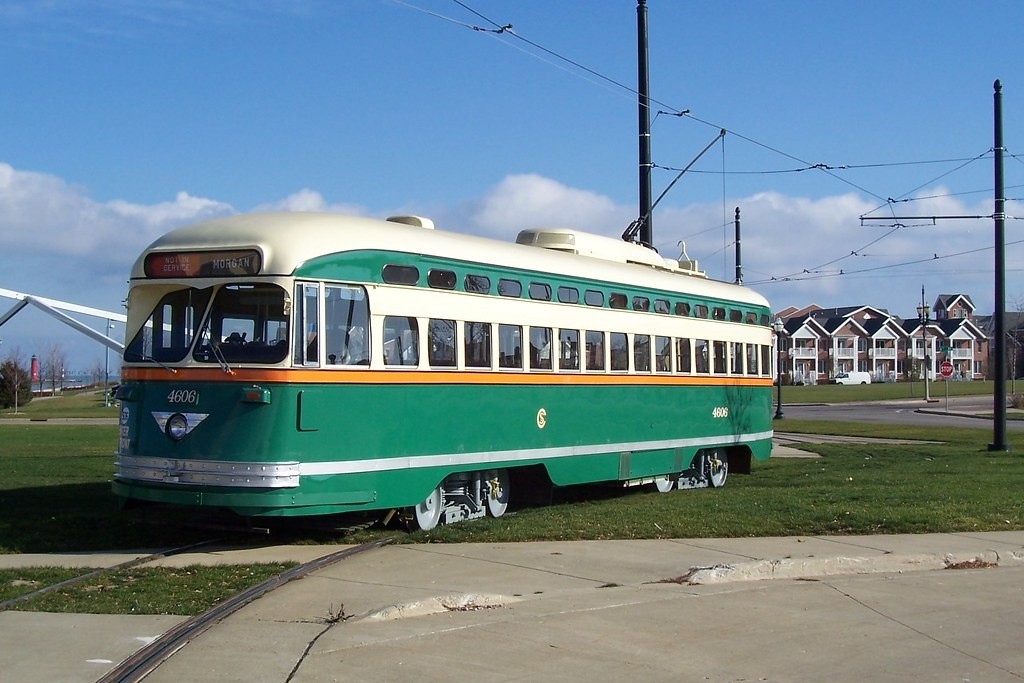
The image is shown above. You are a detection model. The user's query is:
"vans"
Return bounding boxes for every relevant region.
[828,372,872,385]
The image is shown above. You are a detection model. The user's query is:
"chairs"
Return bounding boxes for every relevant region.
[307,329,350,364]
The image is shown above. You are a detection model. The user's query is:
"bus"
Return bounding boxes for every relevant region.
[107,212,779,532]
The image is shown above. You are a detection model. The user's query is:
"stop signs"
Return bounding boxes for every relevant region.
[940,362,954,377]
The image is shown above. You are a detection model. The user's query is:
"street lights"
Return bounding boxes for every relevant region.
[774,317,785,421]
[916,285,933,401]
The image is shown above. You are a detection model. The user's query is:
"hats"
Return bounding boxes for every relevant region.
[225,333,245,342]
